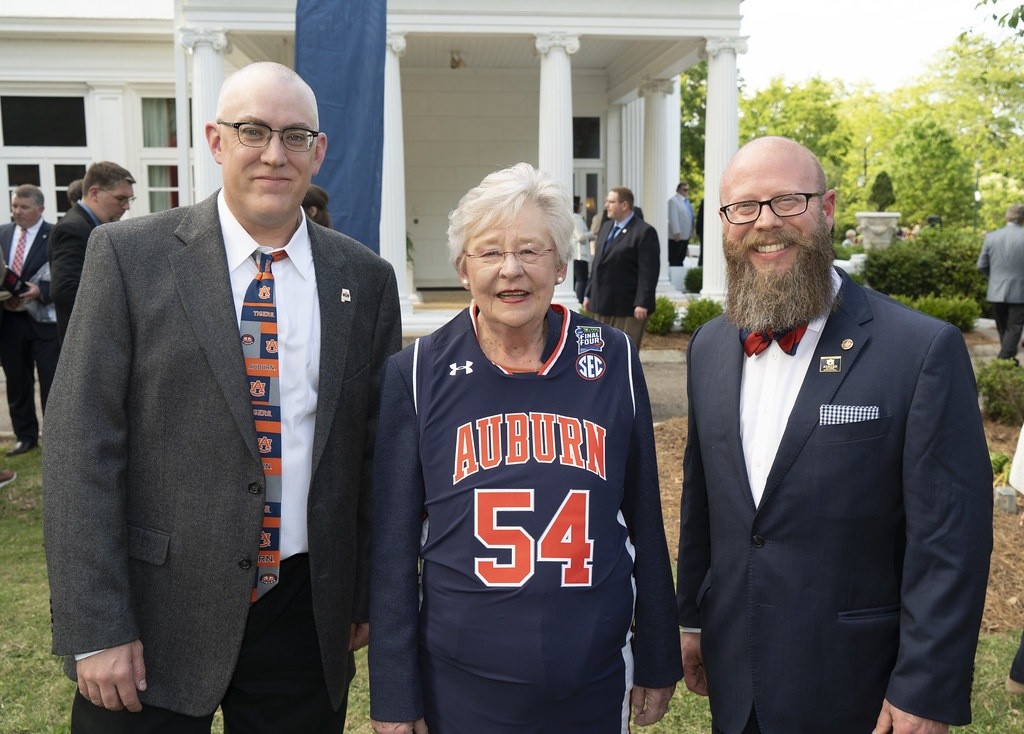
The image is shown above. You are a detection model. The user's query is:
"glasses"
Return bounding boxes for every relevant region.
[719,192,825,225]
[465,242,557,266]
[216,119,319,152]
[99,187,136,207]
[681,189,689,191]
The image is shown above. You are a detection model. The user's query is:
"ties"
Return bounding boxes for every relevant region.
[604,226,619,252]
[6,229,27,308]
[240,252,289,604]
[684,199,693,223]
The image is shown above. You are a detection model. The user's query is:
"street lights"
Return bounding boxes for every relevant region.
[971,158,985,233]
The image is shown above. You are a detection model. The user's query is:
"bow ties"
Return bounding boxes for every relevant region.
[739,319,810,357]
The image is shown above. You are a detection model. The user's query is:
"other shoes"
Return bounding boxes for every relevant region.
[0,469,17,488]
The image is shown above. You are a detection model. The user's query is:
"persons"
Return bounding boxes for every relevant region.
[365,163,685,734]
[675,135,994,734]
[573,187,660,352]
[0,161,137,488]
[977,205,1024,367]
[42,63,404,734]
[1004,422,1024,693]
[667,182,704,267]
[302,184,331,227]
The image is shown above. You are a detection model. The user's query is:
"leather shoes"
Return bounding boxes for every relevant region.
[6,442,39,458]
